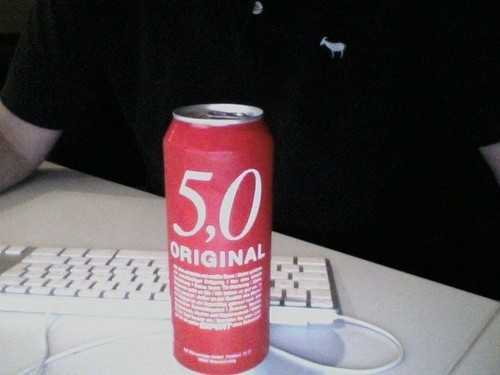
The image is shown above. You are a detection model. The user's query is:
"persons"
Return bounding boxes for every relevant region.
[0,3,499,300]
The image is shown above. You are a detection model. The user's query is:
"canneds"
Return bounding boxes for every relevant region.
[163,103,275,375]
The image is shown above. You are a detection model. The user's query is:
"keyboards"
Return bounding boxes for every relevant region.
[1,241,339,325]
